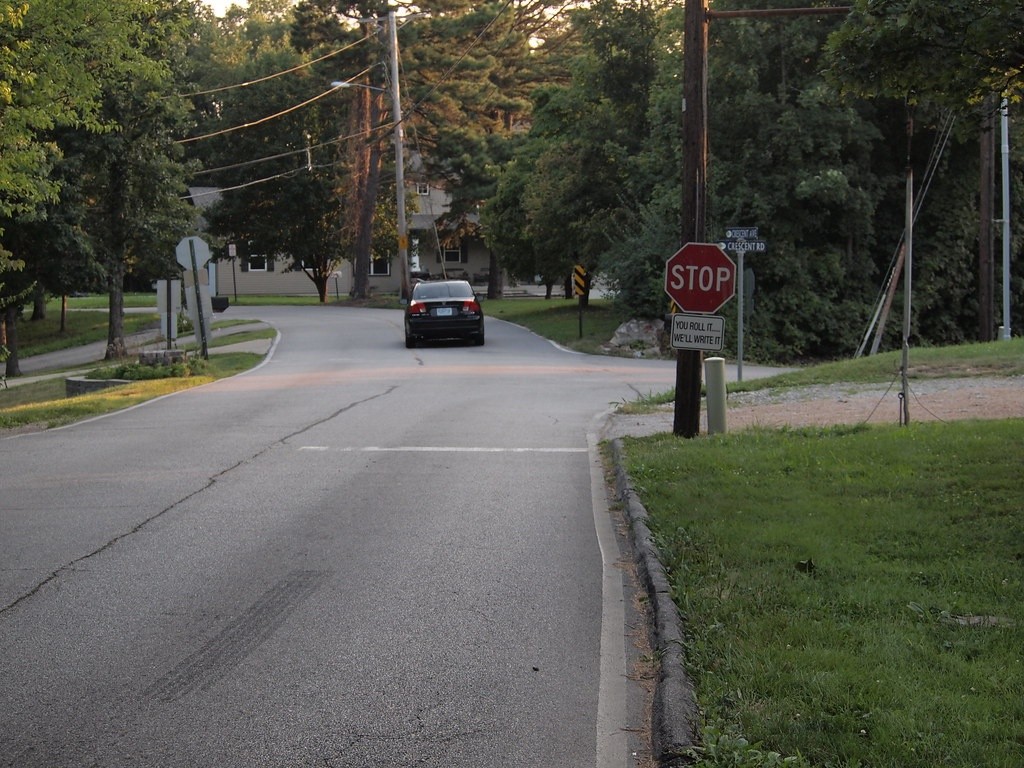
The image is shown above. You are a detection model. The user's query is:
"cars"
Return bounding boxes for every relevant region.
[403,279,485,348]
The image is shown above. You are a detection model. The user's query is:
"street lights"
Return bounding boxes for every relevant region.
[332,78,410,297]
[230,239,239,306]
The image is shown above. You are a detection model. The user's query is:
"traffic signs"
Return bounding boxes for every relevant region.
[719,240,767,252]
[724,227,759,240]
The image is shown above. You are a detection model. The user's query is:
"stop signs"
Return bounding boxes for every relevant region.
[665,241,736,315]
